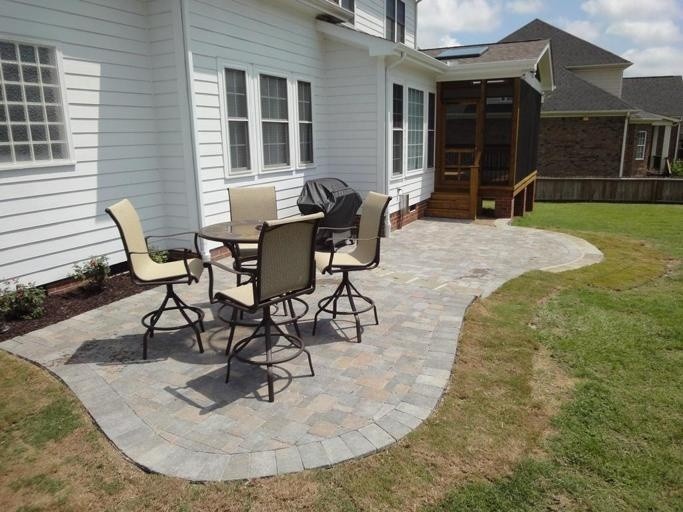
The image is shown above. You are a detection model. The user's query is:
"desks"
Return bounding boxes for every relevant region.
[197,220,309,357]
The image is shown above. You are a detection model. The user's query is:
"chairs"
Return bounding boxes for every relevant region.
[213,184,392,404]
[105,197,206,360]
